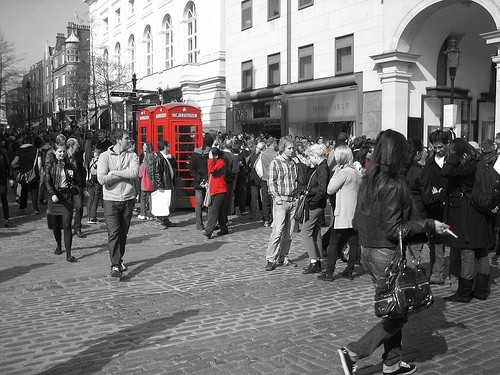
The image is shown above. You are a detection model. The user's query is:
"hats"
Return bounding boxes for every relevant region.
[266,137,279,146]
[468,141,480,150]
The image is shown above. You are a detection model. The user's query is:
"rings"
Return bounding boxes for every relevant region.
[443,230,445,233]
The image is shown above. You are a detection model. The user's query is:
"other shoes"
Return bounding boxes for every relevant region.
[317,271,333,281]
[155,220,176,230]
[264,221,270,227]
[302,261,321,274]
[73,228,83,237]
[54,248,76,262]
[338,270,353,280]
[201,229,228,239]
[265,257,297,271]
[88,218,101,224]
[110,261,127,278]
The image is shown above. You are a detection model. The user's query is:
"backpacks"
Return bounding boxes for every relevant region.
[463,158,500,215]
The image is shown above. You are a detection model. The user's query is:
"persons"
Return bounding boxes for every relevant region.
[55,126,114,238]
[0,126,57,226]
[152,140,177,226]
[290,131,375,281]
[405,130,500,303]
[128,140,156,220]
[45,142,80,262]
[96,128,139,278]
[190,129,277,239]
[338,129,450,375]
[265,137,299,270]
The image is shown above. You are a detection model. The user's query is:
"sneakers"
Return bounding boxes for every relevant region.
[381,360,417,375]
[337,347,358,375]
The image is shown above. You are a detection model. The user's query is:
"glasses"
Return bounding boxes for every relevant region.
[368,150,372,154]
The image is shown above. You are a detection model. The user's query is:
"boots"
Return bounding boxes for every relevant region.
[443,278,474,303]
[473,272,490,300]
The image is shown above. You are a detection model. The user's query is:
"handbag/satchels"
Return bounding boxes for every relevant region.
[293,189,309,225]
[203,184,212,207]
[373,224,434,317]
[150,189,172,217]
[16,167,36,184]
